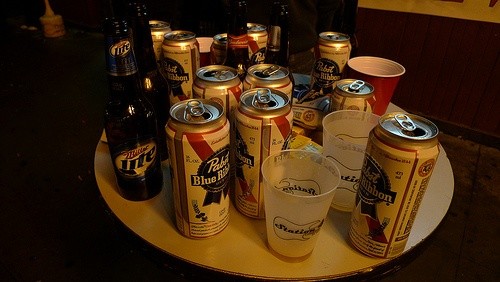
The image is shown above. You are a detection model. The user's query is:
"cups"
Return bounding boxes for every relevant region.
[260,149,341,262]
[320,110,382,213]
[346,55,406,114]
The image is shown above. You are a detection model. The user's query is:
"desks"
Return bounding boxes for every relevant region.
[98,75,455,282]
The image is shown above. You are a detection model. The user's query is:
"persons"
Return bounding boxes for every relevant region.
[285,0,316,74]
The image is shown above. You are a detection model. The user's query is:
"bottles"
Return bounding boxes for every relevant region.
[123,3,168,163]
[262,3,292,71]
[100,14,164,202]
[223,0,251,83]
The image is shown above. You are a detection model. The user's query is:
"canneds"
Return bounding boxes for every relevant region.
[349,112,442,259]
[144,19,374,240]
